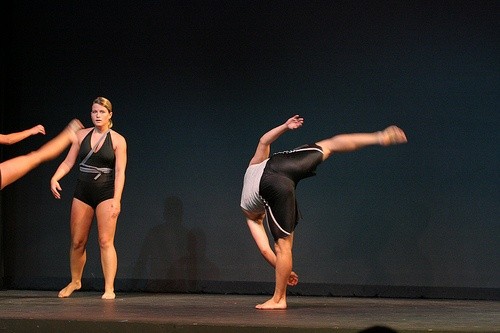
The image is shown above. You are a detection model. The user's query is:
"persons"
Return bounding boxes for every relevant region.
[240,115,408,309]
[51,97,127,300]
[0,119,85,193]
